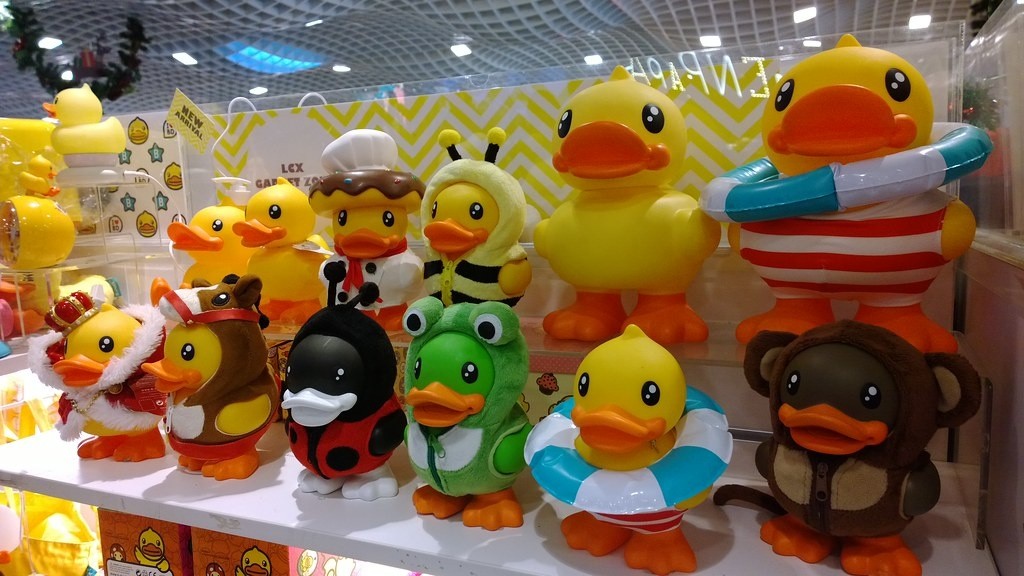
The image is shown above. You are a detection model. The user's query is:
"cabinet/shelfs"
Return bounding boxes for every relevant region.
[0,20,1004,576]
[0,107,186,448]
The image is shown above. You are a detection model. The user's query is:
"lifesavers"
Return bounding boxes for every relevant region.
[522,377,735,516]
[696,118,996,225]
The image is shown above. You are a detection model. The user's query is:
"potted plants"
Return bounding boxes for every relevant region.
[947,76,1007,178]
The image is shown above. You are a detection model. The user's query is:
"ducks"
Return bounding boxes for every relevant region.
[20,32,994,576]
